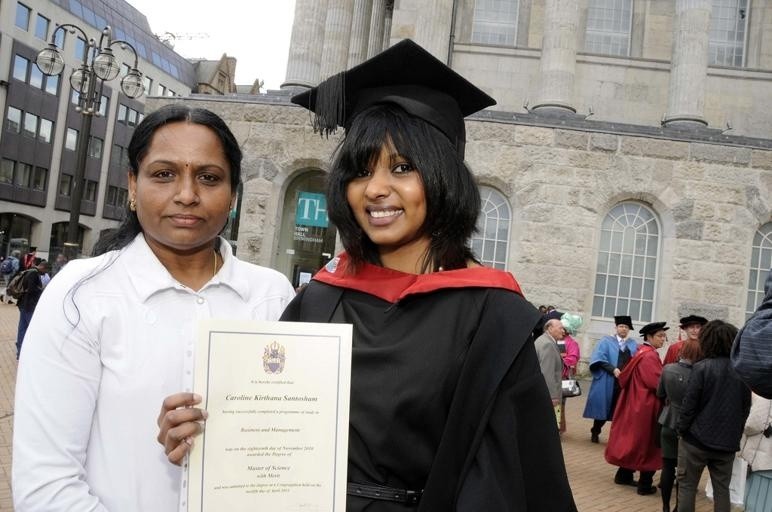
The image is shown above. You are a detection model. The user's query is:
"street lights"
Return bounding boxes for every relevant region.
[35,23,145,262]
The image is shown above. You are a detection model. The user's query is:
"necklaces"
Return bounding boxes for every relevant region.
[213,249,221,281]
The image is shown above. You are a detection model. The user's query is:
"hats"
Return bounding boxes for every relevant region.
[615,316,634,330]
[680,315,708,329]
[639,322,669,336]
[29,247,37,252]
[291,39,496,144]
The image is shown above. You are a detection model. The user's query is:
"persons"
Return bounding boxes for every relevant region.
[533,318,564,432]
[156,36,578,511]
[666,315,708,363]
[582,314,640,443]
[604,321,671,496]
[11,103,295,508]
[672,320,752,511]
[656,338,704,510]
[732,275,767,397]
[740,382,768,507]
[37,257,51,286]
[5,261,52,359]
[556,314,583,432]
[535,303,563,336]
[1,250,21,304]
[20,246,38,270]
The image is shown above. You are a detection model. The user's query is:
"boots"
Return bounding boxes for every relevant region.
[659,458,676,512]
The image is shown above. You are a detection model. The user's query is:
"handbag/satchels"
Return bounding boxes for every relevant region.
[561,366,580,397]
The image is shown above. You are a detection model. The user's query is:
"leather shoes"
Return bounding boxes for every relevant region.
[614,475,638,487]
[637,486,656,496]
[0,295,3,302]
[8,301,15,304]
[592,434,599,442]
[347,481,422,505]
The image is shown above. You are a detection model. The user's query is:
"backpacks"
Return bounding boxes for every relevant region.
[2,257,17,275]
[5,268,42,299]
